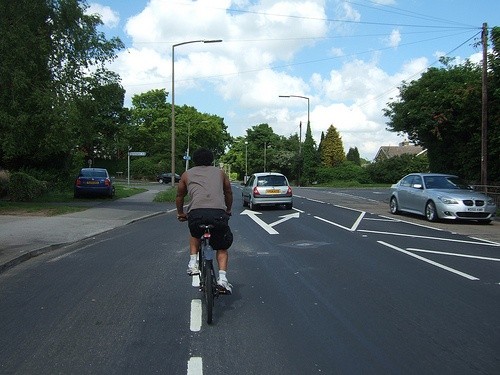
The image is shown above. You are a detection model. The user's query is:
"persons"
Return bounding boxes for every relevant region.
[176,147,234,296]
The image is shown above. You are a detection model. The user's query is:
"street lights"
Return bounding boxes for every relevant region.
[171,40,223,190]
[245,139,248,184]
[186,120,208,171]
[278,95,310,126]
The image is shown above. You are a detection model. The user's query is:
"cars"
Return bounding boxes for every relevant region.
[73,168,115,199]
[241,173,293,210]
[389,173,497,224]
[156,173,178,184]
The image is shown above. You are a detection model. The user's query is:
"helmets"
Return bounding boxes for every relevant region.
[193,148,214,163]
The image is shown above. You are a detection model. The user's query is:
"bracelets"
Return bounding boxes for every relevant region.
[226,210,232,213]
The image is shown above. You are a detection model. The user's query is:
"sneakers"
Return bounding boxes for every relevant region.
[187,265,200,275]
[217,280,232,294]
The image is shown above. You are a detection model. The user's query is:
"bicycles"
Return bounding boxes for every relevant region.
[180,217,227,324]
